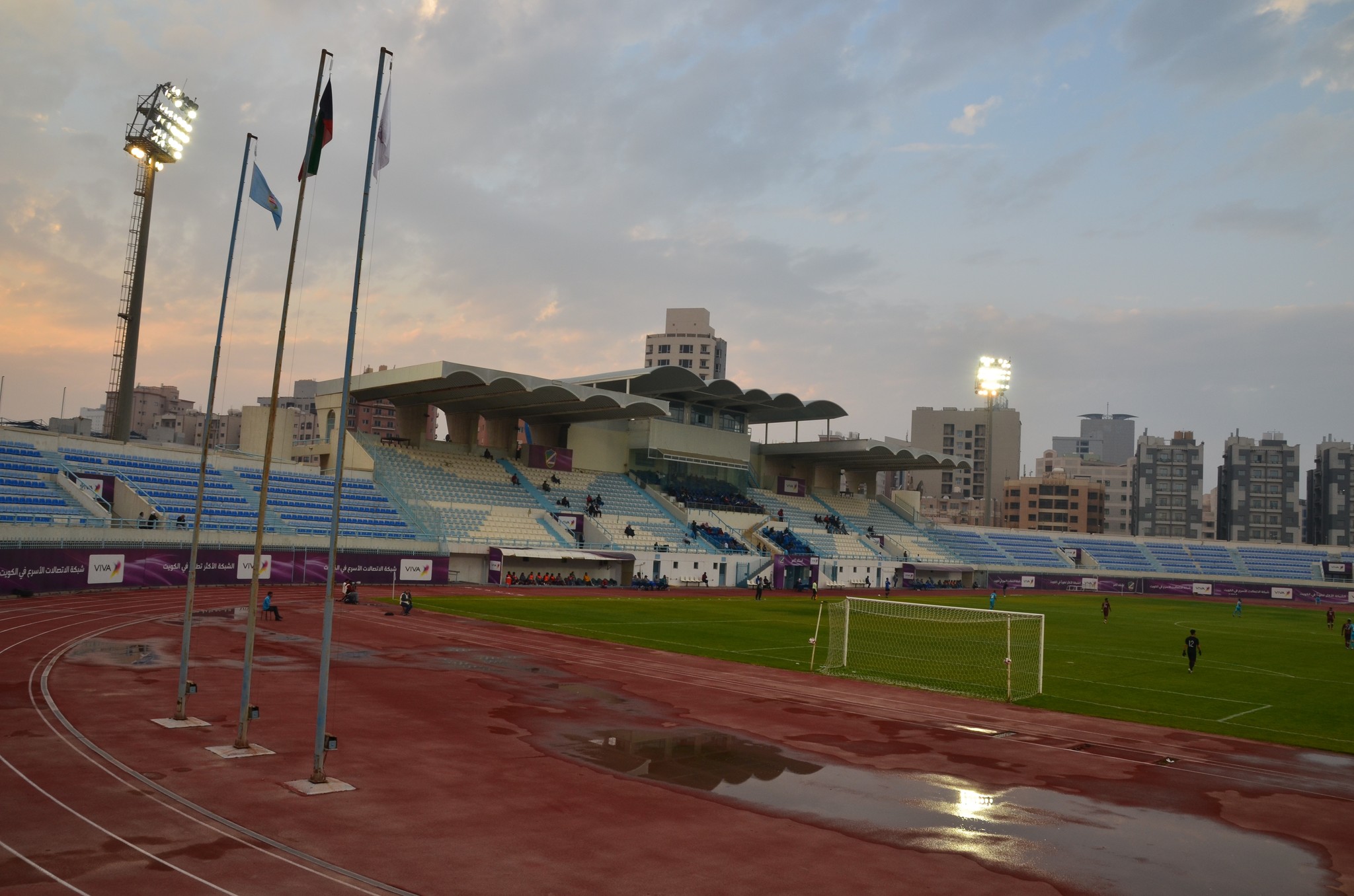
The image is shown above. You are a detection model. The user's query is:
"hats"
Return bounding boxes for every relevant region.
[645,575,648,577]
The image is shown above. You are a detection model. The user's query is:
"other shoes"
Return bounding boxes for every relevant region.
[706,584,708,586]
[278,616,283,619]
[1351,647,1353,649]
[755,599,758,600]
[707,586,709,587]
[758,598,761,600]
[275,619,282,621]
[402,613,406,615]
[407,612,410,615]
[1188,668,1192,672]
[1104,620,1107,623]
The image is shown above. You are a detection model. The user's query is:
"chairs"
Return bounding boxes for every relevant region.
[0,438,1354,590]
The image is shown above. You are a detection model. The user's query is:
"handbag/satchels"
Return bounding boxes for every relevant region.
[815,595,817,600]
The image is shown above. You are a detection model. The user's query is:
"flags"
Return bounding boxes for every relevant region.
[250,163,283,231]
[373,77,391,184]
[298,79,333,181]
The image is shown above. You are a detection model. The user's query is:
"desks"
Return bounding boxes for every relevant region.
[840,491,854,497]
[380,437,411,448]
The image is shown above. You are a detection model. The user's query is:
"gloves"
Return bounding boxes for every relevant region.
[1198,650,1202,656]
[1182,649,1186,656]
[1101,610,1103,613]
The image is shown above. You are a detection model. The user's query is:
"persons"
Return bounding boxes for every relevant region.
[176,514,186,530]
[1182,630,1201,674]
[755,575,817,601]
[1003,582,1008,597]
[702,572,708,587]
[263,591,283,621]
[1101,598,1111,624]
[1233,599,1242,617]
[506,571,592,586]
[632,575,669,591]
[908,577,980,591]
[342,579,359,604]
[136,512,148,529]
[484,447,604,548]
[400,590,413,616]
[988,590,997,610]
[148,511,158,530]
[625,525,635,537]
[1316,596,1321,604]
[654,472,921,562]
[865,572,898,598]
[1327,607,1354,649]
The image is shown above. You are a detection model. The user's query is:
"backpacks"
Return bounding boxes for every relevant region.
[557,500,561,505]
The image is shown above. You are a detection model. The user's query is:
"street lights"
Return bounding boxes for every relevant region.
[975,354,1012,526]
[1098,525,1100,533]
[101,83,198,442]
[1273,535,1277,543]
[1326,536,1327,546]
